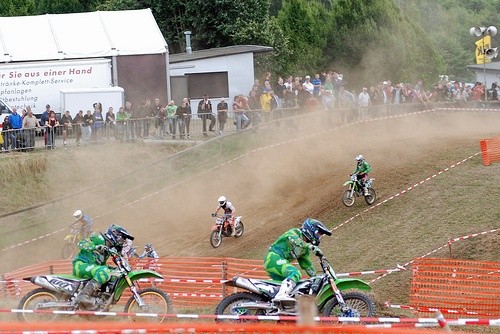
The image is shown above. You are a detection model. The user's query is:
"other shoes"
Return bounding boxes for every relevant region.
[183,137,188,140]
[187,136,191,139]
[63,141,66,145]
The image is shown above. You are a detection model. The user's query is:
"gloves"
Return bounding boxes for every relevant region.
[310,246,323,258]
[212,213,217,216]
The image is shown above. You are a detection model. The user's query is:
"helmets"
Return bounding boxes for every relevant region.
[300,218,332,246]
[104,224,135,249]
[218,195,226,206]
[354,154,363,165]
[144,244,152,253]
[73,210,83,220]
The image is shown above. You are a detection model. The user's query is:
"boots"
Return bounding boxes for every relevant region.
[74,280,102,308]
[273,279,297,307]
[363,187,368,196]
[231,227,236,236]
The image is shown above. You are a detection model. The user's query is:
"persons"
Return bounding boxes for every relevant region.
[73,224,135,308]
[216,97,228,132]
[233,71,500,131]
[140,244,159,262]
[263,218,333,316]
[198,93,216,137]
[73,97,193,147]
[122,239,134,257]
[348,155,370,197]
[69,210,93,238]
[0,105,60,153]
[211,196,237,238]
[61,111,73,149]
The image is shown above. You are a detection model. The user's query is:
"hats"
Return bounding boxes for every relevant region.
[305,76,311,79]
[383,81,387,85]
[46,105,50,108]
[338,77,342,81]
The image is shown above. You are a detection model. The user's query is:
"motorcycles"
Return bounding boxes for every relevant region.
[210,212,244,248]
[214,245,376,327]
[61,225,96,260]
[341,173,376,207]
[16,245,173,327]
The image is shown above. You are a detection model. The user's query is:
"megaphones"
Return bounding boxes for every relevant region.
[477,48,499,58]
[469,26,498,38]
